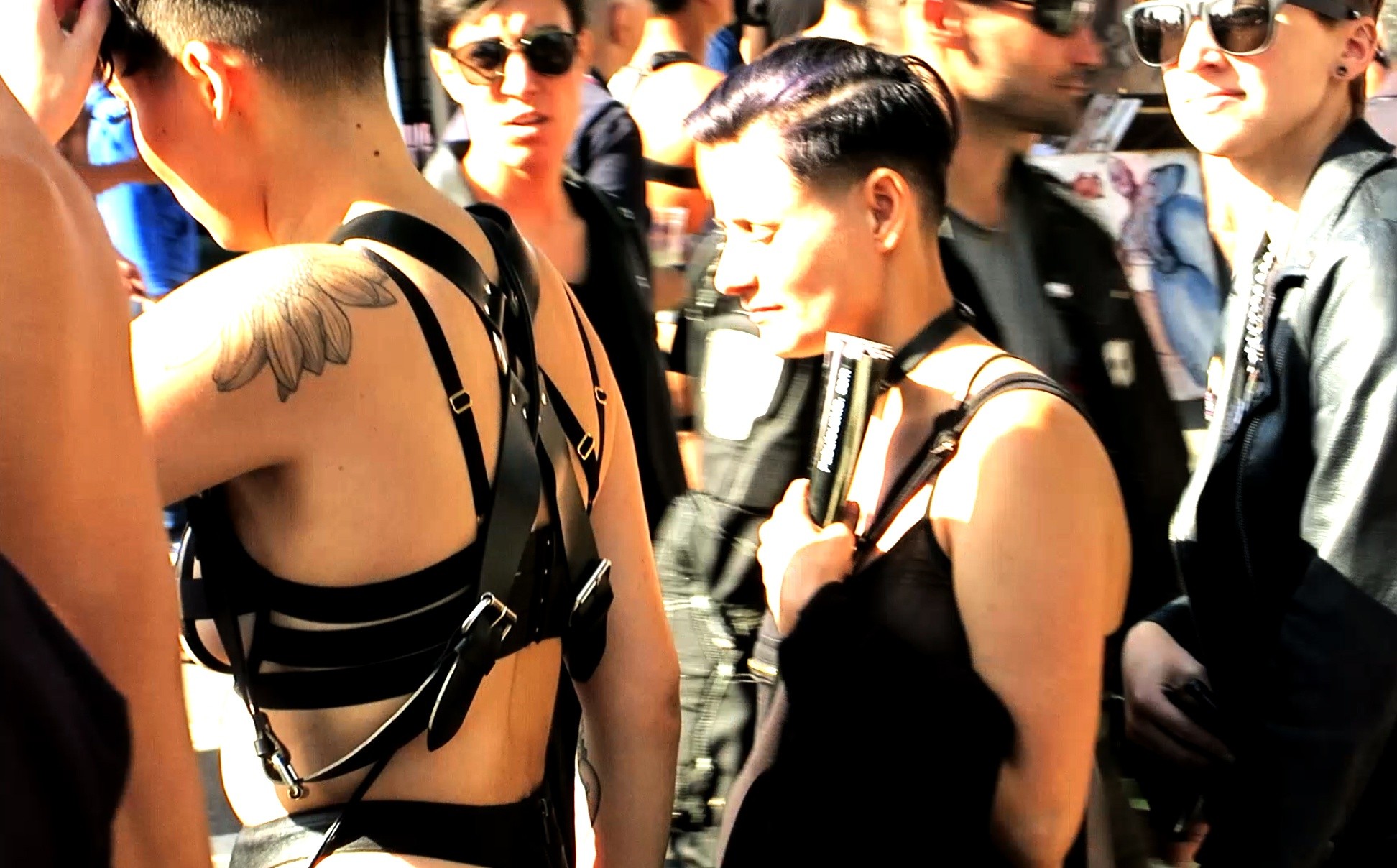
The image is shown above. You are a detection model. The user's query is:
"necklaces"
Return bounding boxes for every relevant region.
[874,299,974,396]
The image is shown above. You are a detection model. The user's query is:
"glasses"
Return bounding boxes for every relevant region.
[1006,0,1119,45]
[1122,0,1351,68]
[436,31,579,87]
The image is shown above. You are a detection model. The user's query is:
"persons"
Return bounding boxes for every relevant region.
[0,0,1397,868]
